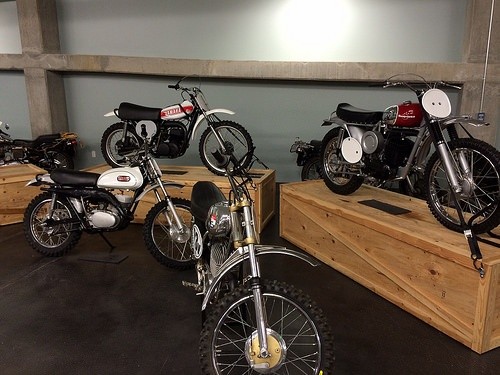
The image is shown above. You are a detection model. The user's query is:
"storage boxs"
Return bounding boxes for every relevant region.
[279,177,500,355]
[78,164,275,235]
[0,162,49,227]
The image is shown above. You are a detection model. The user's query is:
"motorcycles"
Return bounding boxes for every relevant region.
[318,72,500,236]
[290,137,440,200]
[181,149,337,375]
[100,74,255,177]
[23,123,211,269]
[0,121,78,173]
